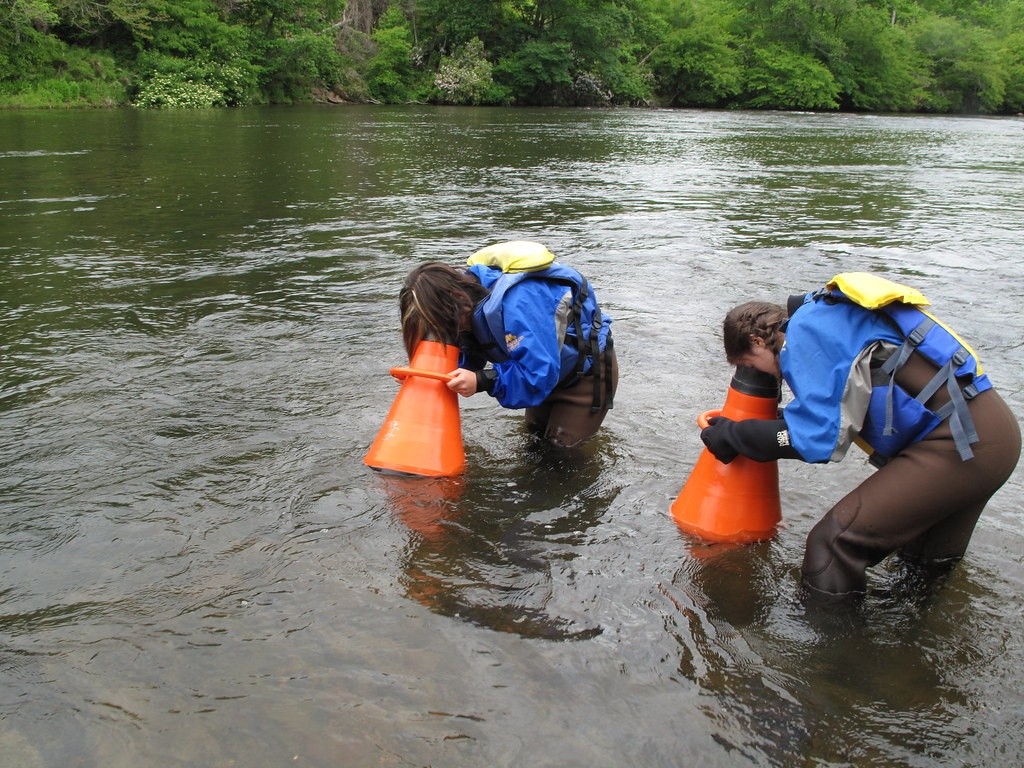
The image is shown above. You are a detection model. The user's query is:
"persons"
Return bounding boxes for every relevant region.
[400,241,618,447]
[700,272,1021,603]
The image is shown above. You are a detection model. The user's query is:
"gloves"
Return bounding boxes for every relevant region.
[700,417,806,464]
